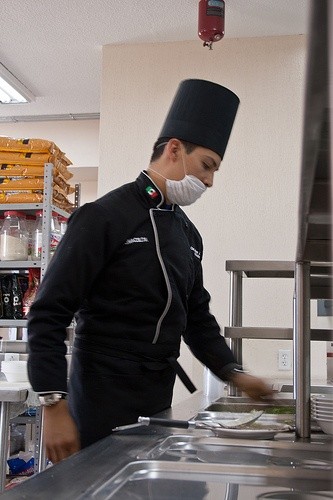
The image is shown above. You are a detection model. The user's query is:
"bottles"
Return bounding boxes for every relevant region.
[0,211,36,261]
[49,212,68,257]
[32,210,43,260]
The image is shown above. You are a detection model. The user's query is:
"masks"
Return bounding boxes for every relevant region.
[165,174,208,206]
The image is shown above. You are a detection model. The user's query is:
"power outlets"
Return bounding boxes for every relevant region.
[277,349,292,370]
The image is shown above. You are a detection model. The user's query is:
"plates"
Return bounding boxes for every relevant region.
[261,392,324,405]
[208,421,291,439]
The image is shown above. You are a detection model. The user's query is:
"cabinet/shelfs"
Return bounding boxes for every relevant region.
[0,164,84,494]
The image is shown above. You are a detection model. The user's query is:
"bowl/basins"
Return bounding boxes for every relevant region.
[311,397,333,434]
[1,361,29,382]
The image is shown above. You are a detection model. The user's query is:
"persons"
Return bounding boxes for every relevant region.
[26,79,280,466]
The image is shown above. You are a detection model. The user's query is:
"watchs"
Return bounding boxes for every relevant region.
[36,393,66,408]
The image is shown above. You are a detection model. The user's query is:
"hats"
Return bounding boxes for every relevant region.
[159,79,240,161]
